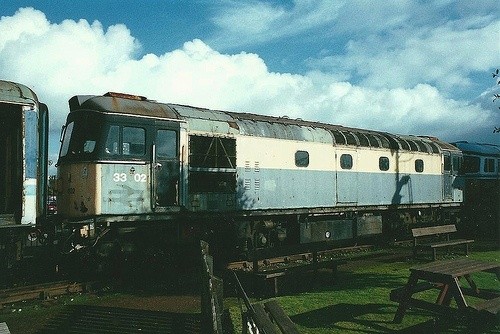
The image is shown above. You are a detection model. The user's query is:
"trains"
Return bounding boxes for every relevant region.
[0,81,500,279]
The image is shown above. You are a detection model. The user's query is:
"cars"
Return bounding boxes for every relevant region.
[48,195,56,214]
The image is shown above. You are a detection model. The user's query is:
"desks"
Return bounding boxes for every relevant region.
[394,261,500,334]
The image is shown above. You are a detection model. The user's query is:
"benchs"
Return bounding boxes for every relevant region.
[229,267,302,334]
[387,279,444,323]
[433,295,499,334]
[410,223,475,261]
